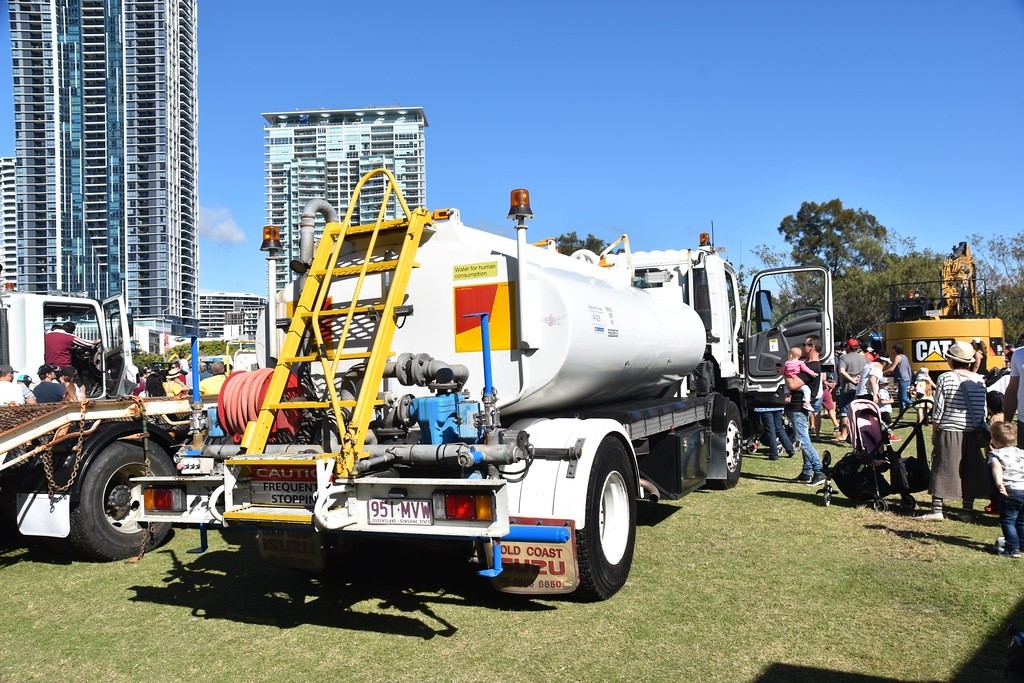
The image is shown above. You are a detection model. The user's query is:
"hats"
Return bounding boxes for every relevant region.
[946,341,976,363]
[846,338,860,349]
[917,368,932,381]
[17,375,33,382]
[36,365,54,375]
[166,369,182,378]
[879,377,889,384]
[0,364,18,374]
[973,337,983,346]
[878,355,892,365]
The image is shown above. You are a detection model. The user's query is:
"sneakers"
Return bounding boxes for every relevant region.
[999,548,1020,558]
[807,472,830,486]
[785,392,791,399]
[889,435,901,441]
[803,402,816,412]
[790,474,811,484]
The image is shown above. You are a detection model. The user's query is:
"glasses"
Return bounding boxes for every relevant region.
[172,366,176,369]
[803,342,810,346]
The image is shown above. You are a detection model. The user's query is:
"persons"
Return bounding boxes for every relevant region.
[33,364,69,402]
[132,351,228,398]
[0,364,37,406]
[17,374,32,388]
[45,321,101,403]
[988,421,1024,557]
[753,329,1024,521]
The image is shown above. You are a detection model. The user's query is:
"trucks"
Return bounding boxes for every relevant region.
[0,283,218,562]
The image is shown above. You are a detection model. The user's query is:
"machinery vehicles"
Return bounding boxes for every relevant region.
[129,167,835,603]
[883,241,1006,384]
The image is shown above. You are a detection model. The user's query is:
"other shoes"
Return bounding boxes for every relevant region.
[834,426,840,431]
[958,513,972,521]
[914,511,944,521]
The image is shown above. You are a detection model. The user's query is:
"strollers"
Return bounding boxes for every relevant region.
[816,399,936,513]
[746,414,802,455]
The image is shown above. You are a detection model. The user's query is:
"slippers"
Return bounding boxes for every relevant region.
[829,436,846,441]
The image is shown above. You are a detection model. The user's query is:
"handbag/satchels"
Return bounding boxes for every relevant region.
[831,445,890,504]
[974,420,991,448]
[887,420,930,494]
